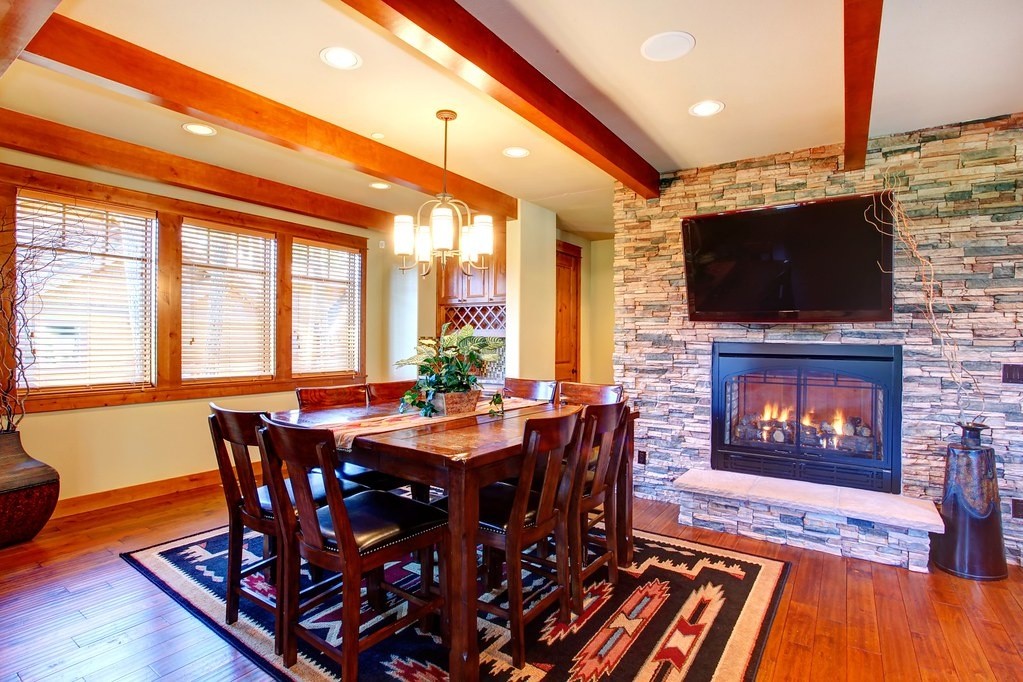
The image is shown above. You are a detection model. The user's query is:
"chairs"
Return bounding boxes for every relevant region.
[208,376,640,682]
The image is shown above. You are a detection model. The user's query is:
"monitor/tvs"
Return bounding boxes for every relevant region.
[682,191,895,323]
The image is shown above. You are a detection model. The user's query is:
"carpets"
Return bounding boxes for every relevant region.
[121,517,791,682]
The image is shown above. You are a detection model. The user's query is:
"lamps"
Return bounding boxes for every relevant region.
[394,111,493,279]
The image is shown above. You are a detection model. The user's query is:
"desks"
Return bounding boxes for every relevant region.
[270,392,640,682]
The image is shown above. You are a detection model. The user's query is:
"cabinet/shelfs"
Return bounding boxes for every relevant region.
[435,210,505,305]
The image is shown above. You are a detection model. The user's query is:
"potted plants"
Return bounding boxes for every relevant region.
[0,189,119,549]
[863,165,1009,581]
[393,320,514,418]
